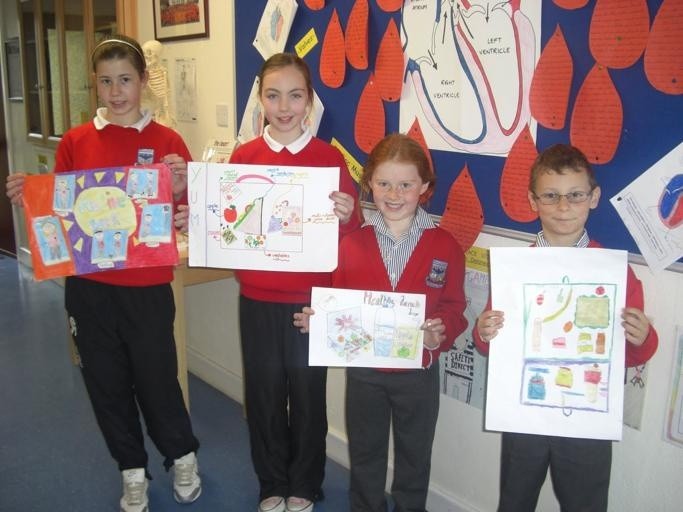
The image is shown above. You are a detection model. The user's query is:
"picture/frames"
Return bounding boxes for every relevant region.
[2,38,26,103]
[151,0,210,43]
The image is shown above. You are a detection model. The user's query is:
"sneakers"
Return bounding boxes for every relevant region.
[116,467,151,512]
[171,451,203,505]
[256,493,315,512]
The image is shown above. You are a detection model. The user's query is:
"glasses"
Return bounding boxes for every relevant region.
[528,188,594,205]
[365,177,424,194]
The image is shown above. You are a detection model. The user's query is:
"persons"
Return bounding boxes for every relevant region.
[472,144,660,512]
[171,52,367,512]
[290,134,469,512]
[3,33,204,512]
